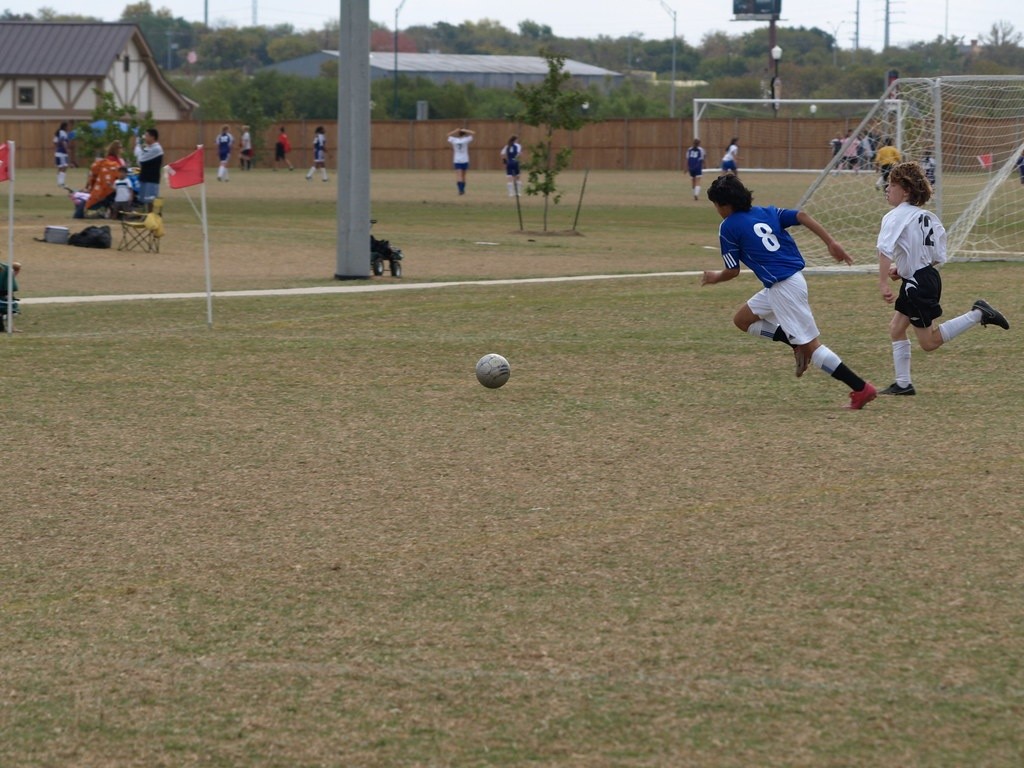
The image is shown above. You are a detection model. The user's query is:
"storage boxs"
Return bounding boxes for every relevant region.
[45,227,69,243]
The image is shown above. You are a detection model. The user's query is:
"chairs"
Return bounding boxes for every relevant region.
[119,197,164,254]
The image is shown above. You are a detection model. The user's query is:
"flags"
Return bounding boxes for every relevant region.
[977,152,992,168]
[0,143,10,181]
[163,145,205,189]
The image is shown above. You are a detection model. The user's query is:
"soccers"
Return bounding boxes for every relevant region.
[475,353,510,389]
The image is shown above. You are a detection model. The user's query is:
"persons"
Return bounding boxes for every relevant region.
[239,125,251,171]
[215,124,234,182]
[877,162,1009,396]
[272,126,294,171]
[500,135,523,196]
[54,122,165,222]
[701,173,878,409]
[445,128,476,194]
[829,130,1024,206]
[684,138,706,200]
[305,126,331,181]
[721,136,740,178]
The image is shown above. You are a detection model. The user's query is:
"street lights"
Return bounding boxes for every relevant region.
[827,19,846,39]
[771,45,782,119]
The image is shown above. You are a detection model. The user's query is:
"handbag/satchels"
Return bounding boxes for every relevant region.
[72,226,111,248]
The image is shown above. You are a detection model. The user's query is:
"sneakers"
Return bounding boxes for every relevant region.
[794,344,812,377]
[878,383,916,396]
[849,385,877,410]
[972,298,1010,330]
